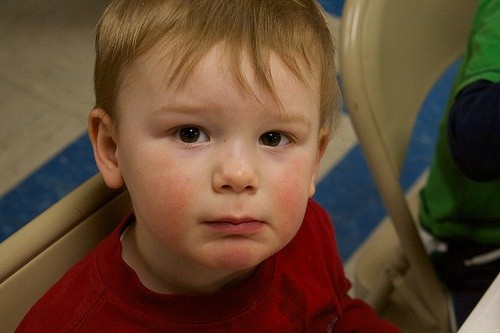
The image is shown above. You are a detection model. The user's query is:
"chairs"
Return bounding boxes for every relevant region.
[1,159,142,333]
[337,0,485,333]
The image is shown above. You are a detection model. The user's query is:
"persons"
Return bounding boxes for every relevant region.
[413,0,500,333]
[15,1,404,332]
